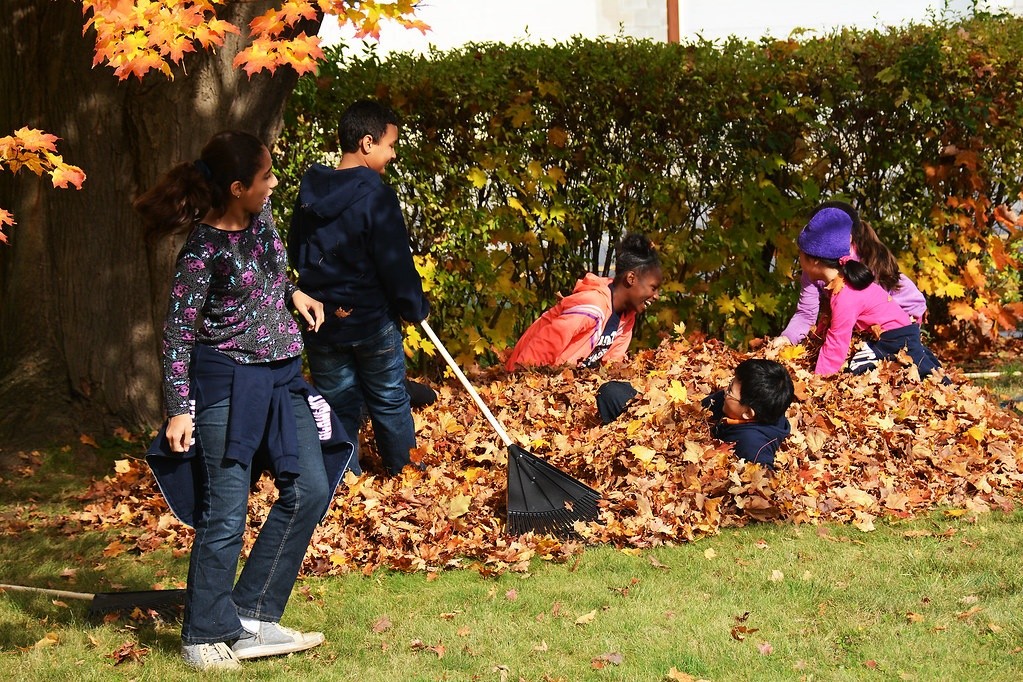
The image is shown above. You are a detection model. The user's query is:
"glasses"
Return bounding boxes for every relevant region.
[724,378,740,402]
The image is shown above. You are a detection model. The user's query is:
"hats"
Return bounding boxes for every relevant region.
[797,207,853,258]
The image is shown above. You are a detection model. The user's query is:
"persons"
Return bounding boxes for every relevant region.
[504,233,664,373]
[796,207,949,388]
[595,358,795,473]
[287,98,432,481]
[766,200,953,388]
[132,128,356,674]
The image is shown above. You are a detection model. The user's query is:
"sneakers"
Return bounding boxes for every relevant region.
[181,640,242,670]
[230,619,325,660]
